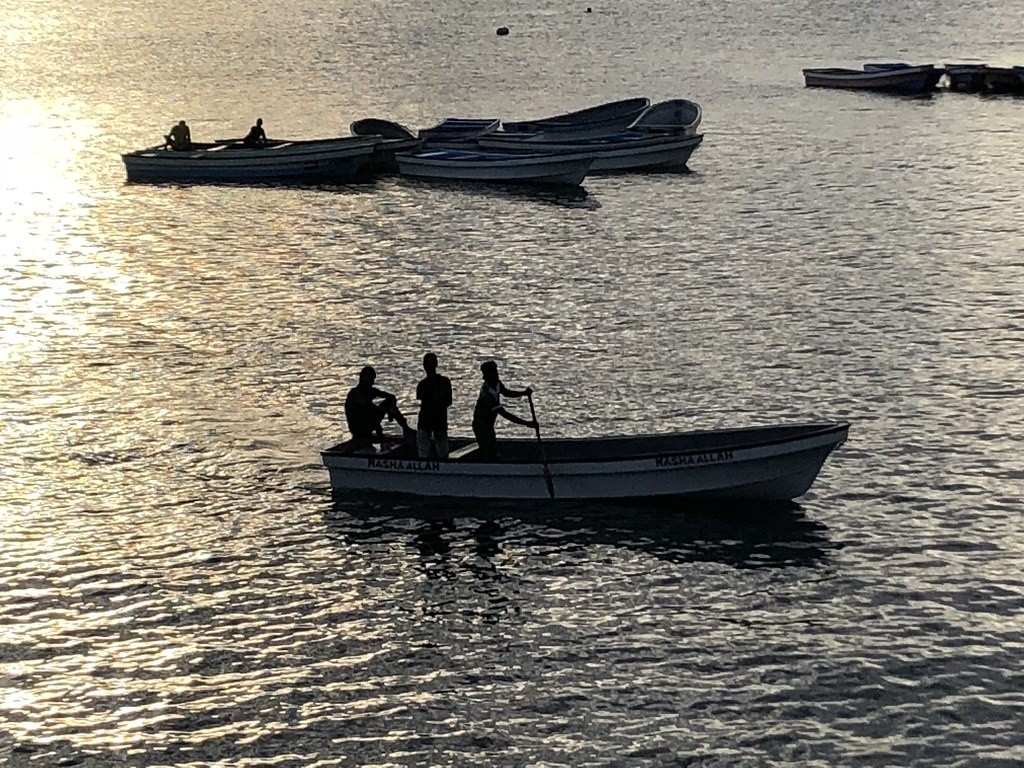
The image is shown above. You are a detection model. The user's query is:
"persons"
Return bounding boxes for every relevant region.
[162,120,193,150]
[415,352,453,464]
[243,118,267,151]
[471,360,539,465]
[344,366,418,451]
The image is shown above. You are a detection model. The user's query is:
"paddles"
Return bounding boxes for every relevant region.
[525,386,555,499]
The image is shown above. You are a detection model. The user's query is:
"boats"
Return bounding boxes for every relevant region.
[319,419,852,510]
[501,97,650,133]
[862,62,945,89]
[944,63,1024,96]
[802,63,935,93]
[625,99,702,135]
[120,118,705,188]
